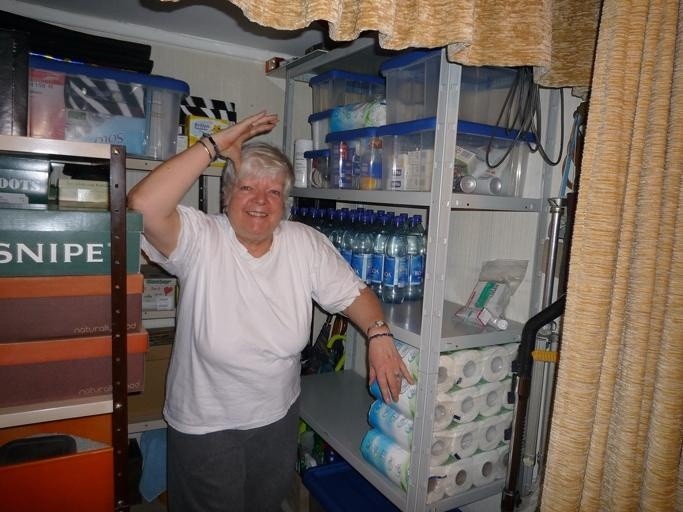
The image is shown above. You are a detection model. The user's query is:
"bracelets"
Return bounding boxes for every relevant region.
[199,132,220,155]
[366,333,394,342]
[195,139,213,161]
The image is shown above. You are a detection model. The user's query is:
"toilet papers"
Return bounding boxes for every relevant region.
[359,339,522,507]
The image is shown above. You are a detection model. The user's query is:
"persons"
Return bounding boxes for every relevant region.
[124,108,414,512]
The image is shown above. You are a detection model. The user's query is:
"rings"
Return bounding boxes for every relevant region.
[395,372,403,380]
[248,123,254,128]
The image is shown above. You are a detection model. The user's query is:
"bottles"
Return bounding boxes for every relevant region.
[453,176,503,195]
[289,206,424,305]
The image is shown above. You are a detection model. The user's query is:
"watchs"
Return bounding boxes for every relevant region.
[365,317,389,337]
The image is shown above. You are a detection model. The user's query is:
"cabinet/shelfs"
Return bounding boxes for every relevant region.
[285,37,559,512]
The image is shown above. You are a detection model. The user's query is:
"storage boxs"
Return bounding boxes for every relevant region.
[300,463,401,512]
[303,45,539,198]
[0,52,190,512]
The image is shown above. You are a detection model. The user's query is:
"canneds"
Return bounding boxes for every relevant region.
[453,175,477,194]
[474,176,503,195]
